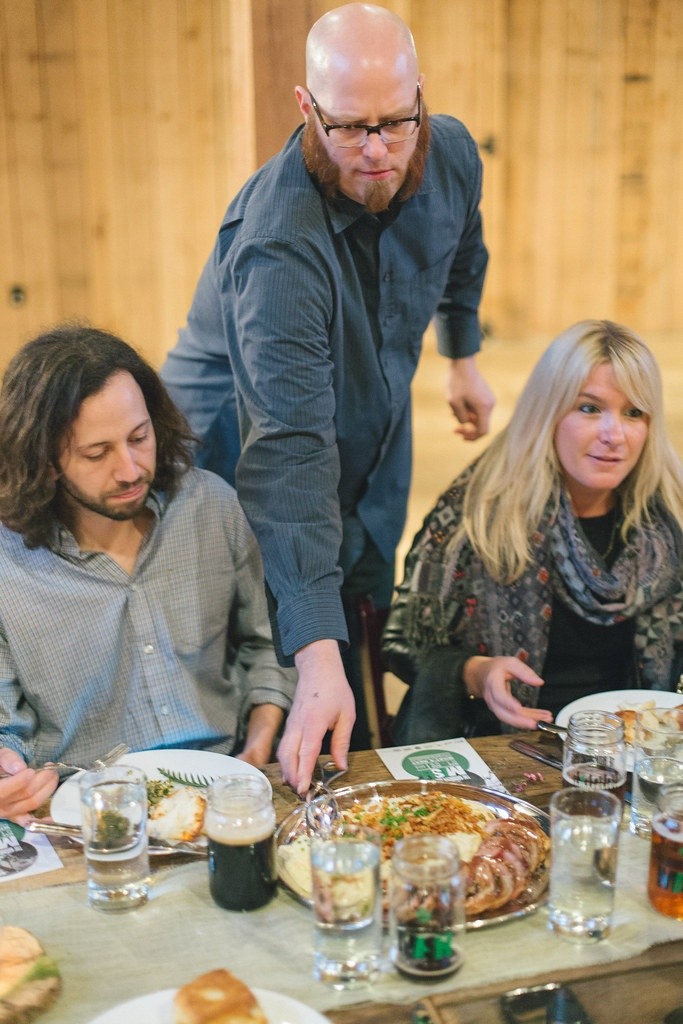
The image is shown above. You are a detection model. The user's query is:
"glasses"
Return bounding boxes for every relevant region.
[308,84,421,148]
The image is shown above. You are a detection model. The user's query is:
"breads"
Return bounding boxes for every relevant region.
[174,968,271,1024]
[605,709,653,745]
[0,924,60,1024]
[662,704,683,731]
[144,786,206,842]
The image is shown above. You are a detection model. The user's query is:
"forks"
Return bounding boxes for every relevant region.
[0,743,132,775]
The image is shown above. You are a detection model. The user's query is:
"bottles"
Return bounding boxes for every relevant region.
[207,774,277,912]
[561,711,627,810]
[647,782,683,920]
[388,836,467,981]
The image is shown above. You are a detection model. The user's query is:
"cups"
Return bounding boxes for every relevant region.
[309,827,381,987]
[549,787,622,945]
[77,765,152,911]
[633,708,683,841]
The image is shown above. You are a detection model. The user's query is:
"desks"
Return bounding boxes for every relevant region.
[0,726,683,1024]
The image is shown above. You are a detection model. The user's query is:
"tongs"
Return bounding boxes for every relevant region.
[304,762,347,833]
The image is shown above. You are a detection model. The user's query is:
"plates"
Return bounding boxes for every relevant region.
[49,748,273,856]
[556,690,683,756]
[273,779,550,932]
[90,989,332,1024]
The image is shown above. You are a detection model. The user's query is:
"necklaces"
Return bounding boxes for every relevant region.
[602,506,618,559]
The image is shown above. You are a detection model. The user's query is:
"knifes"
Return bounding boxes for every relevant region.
[509,740,632,805]
[26,821,208,855]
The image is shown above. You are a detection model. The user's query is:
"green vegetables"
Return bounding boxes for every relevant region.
[338,798,446,845]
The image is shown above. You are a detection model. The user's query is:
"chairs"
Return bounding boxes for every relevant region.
[354,591,415,750]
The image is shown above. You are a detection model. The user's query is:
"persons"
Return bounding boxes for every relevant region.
[155,2,496,794]
[380,320,683,746]
[0,323,298,824]
